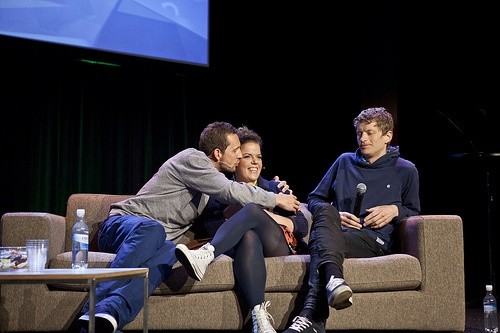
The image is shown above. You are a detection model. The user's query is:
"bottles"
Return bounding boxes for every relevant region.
[70,208,89,270]
[483,285,498,333]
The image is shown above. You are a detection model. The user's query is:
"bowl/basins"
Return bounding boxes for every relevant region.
[0,246,28,273]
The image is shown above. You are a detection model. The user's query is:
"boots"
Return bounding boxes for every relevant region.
[174,241,214,281]
[251,301,277,333]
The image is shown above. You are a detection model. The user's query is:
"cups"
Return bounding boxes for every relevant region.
[26,239,51,273]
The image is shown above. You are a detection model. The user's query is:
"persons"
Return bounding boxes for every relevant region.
[174,126,309,333]
[284,107,421,333]
[77,121,301,333]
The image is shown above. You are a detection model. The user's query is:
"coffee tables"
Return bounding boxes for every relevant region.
[0,267,149,333]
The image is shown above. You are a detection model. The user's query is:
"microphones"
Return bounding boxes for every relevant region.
[219,160,233,168]
[353,182,367,217]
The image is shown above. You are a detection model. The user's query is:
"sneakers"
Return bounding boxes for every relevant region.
[79,305,118,333]
[281,316,325,333]
[325,274,353,310]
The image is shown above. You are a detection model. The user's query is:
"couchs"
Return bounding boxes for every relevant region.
[0,192,466,333]
